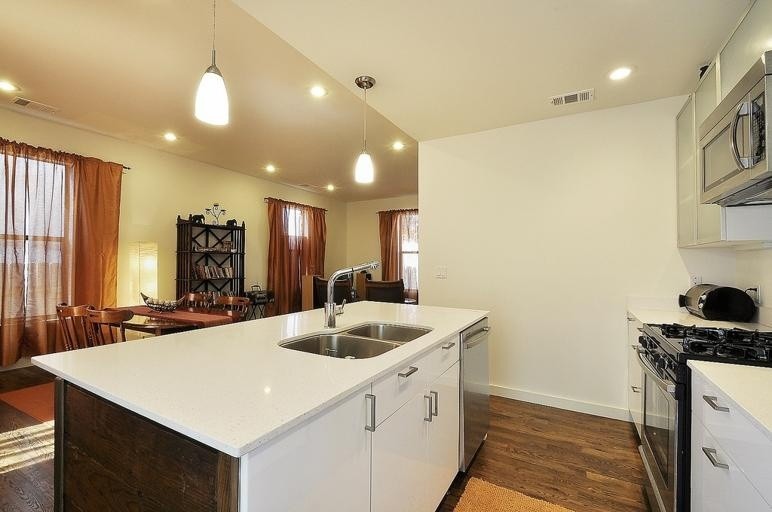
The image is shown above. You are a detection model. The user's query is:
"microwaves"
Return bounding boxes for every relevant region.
[696,50,772,208]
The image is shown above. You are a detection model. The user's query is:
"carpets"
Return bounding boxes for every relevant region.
[454,475,576,512]
[1,378,54,424]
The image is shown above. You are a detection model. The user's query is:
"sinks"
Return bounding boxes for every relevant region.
[279,335,402,360]
[346,323,433,341]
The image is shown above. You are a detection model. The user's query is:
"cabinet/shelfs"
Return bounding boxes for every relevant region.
[239,381,371,512]
[627,309,645,439]
[675,54,772,250]
[690,358,772,511]
[174,214,247,300]
[371,333,461,512]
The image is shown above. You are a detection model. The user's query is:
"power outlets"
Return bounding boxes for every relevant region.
[753,285,761,303]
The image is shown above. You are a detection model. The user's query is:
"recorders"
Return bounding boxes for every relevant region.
[244,285,275,305]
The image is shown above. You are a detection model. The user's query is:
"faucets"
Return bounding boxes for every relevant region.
[328,262,380,302]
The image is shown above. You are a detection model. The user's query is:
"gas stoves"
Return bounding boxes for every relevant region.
[638,322,772,384]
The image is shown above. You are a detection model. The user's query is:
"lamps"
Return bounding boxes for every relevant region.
[195,2,229,127]
[354,75,376,184]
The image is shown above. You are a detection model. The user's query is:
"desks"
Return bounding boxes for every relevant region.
[404,297,416,303]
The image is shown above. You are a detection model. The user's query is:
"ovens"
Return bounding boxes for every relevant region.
[631,343,691,512]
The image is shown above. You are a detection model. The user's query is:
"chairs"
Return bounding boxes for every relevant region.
[364,279,405,304]
[312,275,353,308]
[55,292,251,351]
